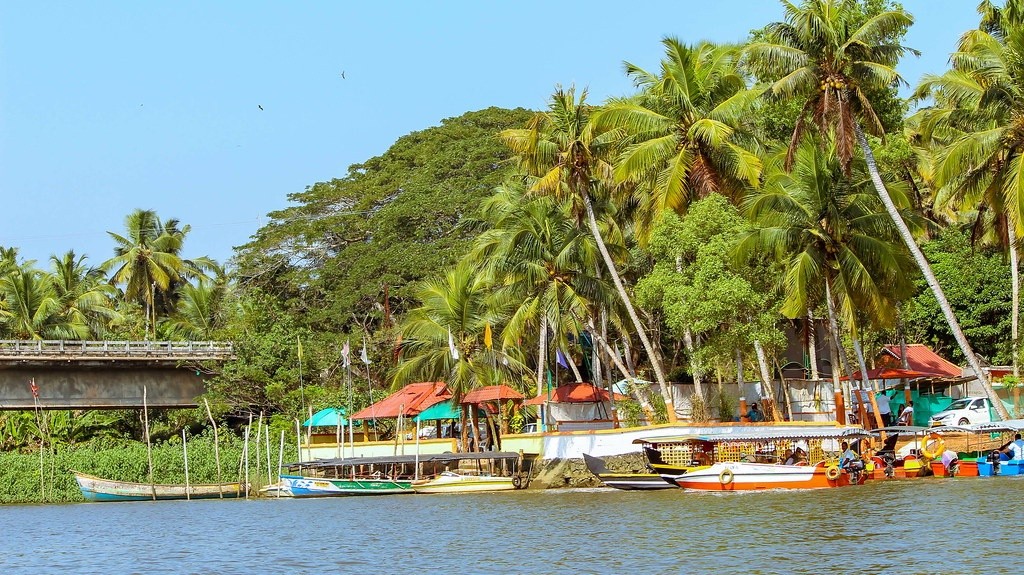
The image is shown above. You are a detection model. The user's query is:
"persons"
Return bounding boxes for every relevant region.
[895,401,914,426]
[877,390,893,432]
[785,449,801,465]
[488,420,501,451]
[742,403,765,421]
[994,434,1024,460]
[842,442,856,472]
[449,422,456,437]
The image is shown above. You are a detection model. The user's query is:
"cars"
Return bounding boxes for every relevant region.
[927,396,1013,426]
[407,421,537,444]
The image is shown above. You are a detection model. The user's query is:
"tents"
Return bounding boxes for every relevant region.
[523,382,634,432]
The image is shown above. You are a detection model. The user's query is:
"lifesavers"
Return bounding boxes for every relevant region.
[512,476,521,486]
[921,434,946,459]
[719,469,734,485]
[825,465,841,480]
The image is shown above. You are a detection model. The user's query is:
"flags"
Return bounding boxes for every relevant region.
[298,341,303,359]
[360,346,372,364]
[449,337,459,359]
[556,349,568,368]
[341,340,349,367]
[484,323,492,351]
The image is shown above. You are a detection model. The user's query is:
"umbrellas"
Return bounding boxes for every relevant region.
[413,398,485,421]
[303,408,373,426]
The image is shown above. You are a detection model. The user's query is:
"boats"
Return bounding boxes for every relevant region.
[579,420,1024,483]
[71,468,253,504]
[276,448,522,497]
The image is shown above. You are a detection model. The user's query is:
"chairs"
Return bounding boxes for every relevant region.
[845,411,911,430]
